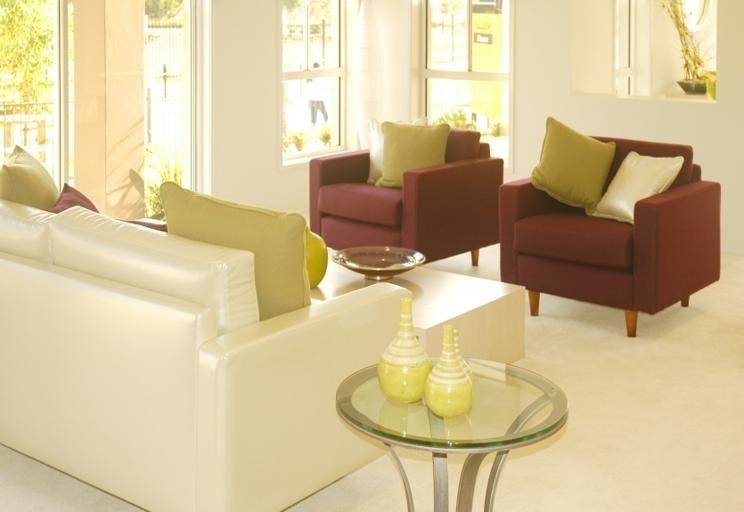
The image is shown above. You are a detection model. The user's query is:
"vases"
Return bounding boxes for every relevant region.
[422,322,474,419]
[375,295,433,404]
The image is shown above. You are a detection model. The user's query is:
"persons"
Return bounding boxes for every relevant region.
[308,62,328,124]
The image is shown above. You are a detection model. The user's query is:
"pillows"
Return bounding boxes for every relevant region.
[590,149,686,226]
[158,179,312,322]
[365,120,386,185]
[0,145,58,211]
[528,116,617,217]
[47,181,99,220]
[374,119,452,188]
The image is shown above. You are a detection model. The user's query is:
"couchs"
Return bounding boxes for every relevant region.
[0,197,416,511]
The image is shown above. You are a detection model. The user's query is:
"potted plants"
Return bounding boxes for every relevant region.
[662,0,707,95]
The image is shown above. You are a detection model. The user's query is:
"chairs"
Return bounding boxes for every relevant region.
[307,129,505,270]
[496,135,723,337]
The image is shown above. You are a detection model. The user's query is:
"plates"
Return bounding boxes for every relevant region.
[333,244,427,282]
